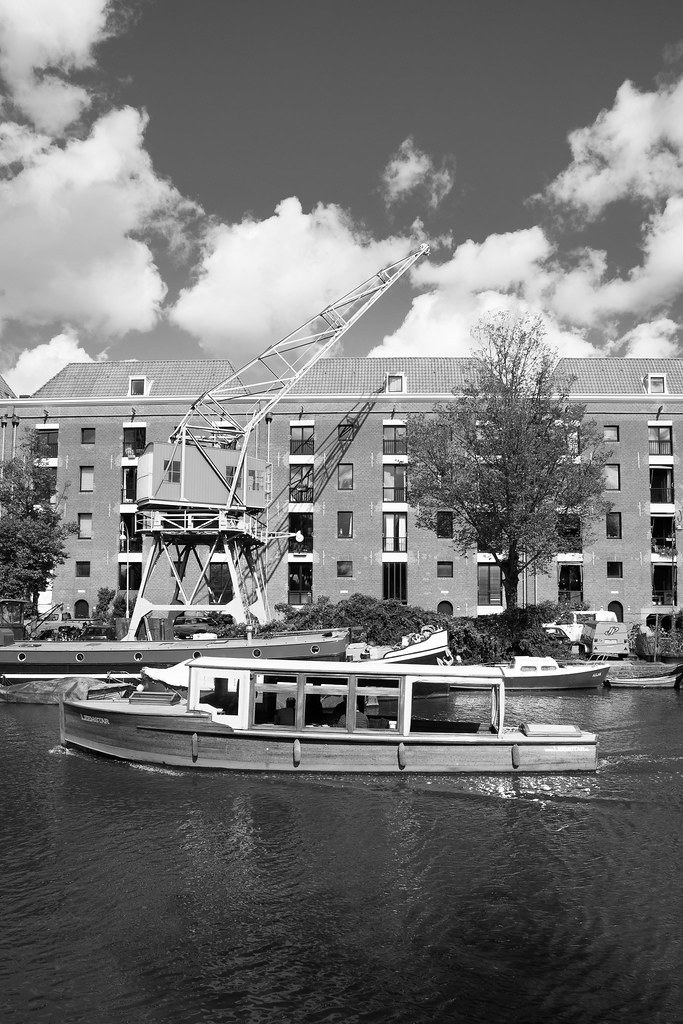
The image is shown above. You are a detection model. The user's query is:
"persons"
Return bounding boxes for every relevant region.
[274,697,295,725]
[337,703,369,728]
[332,695,347,718]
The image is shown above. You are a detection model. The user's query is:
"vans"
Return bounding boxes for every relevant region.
[540,610,617,641]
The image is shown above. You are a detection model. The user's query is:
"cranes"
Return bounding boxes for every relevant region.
[121,241,431,642]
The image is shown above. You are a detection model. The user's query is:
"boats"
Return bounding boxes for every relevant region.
[601,664,683,690]
[59,655,602,775]
[0,672,133,705]
[344,624,611,690]
